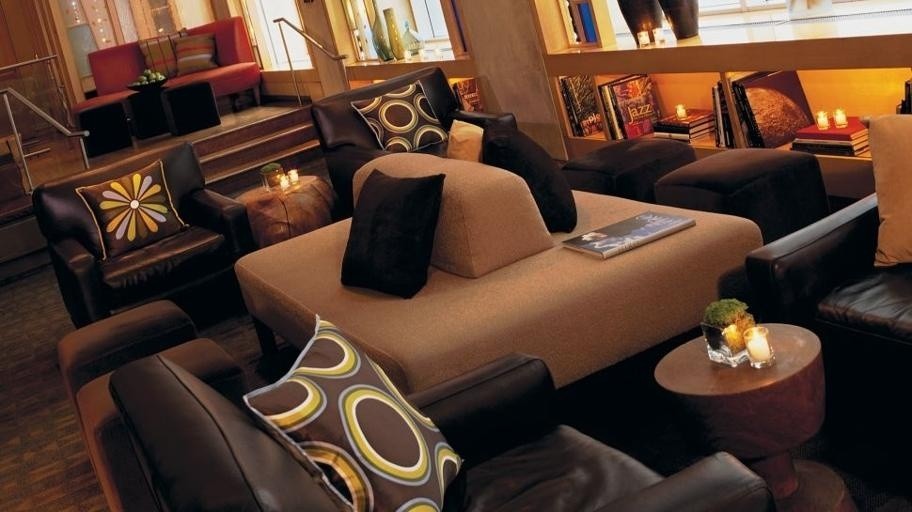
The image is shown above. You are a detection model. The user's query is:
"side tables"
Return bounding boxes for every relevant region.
[234,176,339,248]
[654,323,855,510]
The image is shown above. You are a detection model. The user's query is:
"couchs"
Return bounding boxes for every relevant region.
[72,16,261,125]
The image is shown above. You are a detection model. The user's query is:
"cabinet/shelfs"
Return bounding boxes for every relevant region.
[529,0,911,200]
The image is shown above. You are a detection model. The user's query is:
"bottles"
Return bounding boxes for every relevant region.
[403,20,422,56]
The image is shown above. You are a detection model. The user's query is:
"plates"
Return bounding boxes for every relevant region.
[126,77,168,92]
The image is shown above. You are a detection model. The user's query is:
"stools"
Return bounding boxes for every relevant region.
[561,138,829,246]
[234,186,764,396]
[76,81,221,158]
[57,300,246,511]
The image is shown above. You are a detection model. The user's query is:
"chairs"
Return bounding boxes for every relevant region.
[109,353,777,511]
[310,67,517,211]
[32,140,256,329]
[745,190,911,441]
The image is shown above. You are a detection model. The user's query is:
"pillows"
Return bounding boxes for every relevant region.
[858,113,911,267]
[137,28,217,79]
[75,158,190,259]
[244,314,464,510]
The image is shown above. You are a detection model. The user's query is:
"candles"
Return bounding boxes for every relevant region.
[675,104,684,119]
[635,28,667,49]
[748,326,770,362]
[813,109,850,129]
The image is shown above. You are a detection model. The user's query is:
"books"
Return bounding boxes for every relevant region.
[562,210,696,260]
[453,79,483,114]
[559,71,604,137]
[732,69,876,157]
[597,73,735,150]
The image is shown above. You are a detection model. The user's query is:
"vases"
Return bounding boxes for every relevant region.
[383,6,424,61]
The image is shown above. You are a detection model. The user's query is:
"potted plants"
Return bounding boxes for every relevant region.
[701,298,757,367]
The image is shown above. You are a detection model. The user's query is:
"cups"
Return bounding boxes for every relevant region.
[637,30,651,48]
[742,326,776,369]
[832,108,848,129]
[280,175,290,191]
[288,169,299,186]
[815,111,830,130]
[674,104,687,121]
[652,27,666,45]
